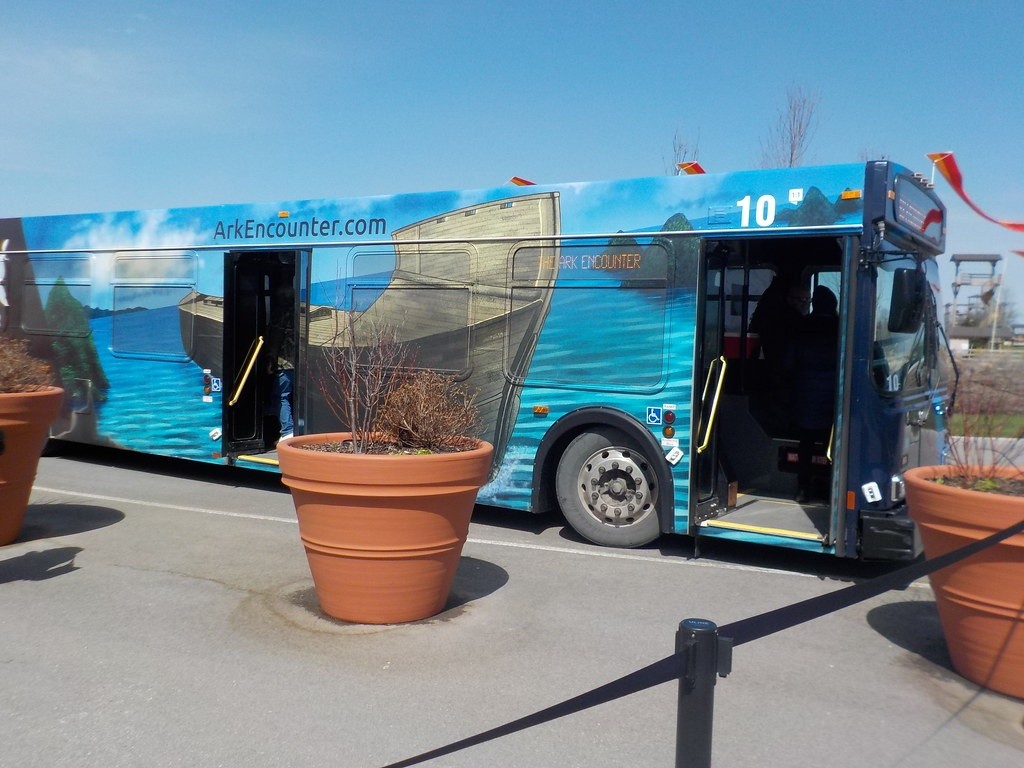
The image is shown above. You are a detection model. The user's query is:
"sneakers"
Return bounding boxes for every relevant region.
[796,485,826,503]
[273,433,294,445]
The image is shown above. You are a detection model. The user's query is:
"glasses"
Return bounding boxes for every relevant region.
[792,295,813,304]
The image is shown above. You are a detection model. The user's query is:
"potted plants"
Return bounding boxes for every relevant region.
[0,327,66,547]
[276,300,495,625]
[902,348,1024,701]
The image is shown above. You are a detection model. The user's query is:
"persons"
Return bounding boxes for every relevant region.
[749,265,840,502]
[265,288,296,446]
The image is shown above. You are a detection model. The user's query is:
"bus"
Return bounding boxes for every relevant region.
[0,160,960,563]
